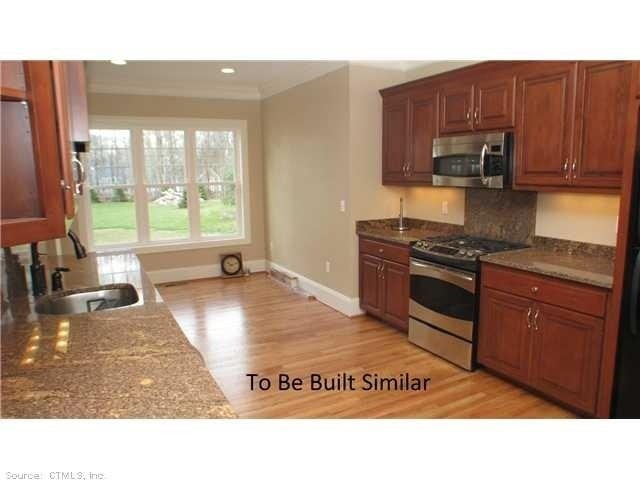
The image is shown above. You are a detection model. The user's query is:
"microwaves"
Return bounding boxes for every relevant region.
[432,141,508,189]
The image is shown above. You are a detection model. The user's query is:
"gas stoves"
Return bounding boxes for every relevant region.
[413,233,533,259]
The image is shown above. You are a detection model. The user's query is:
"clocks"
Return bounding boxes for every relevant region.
[218,250,246,278]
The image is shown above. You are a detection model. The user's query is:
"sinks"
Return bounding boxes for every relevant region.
[33,282,139,317]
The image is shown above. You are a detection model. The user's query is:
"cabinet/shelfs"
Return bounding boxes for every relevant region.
[0,60,90,249]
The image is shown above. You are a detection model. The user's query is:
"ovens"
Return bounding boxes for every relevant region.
[409,259,479,371]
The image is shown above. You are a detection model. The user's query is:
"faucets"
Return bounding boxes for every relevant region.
[29,228,87,296]
